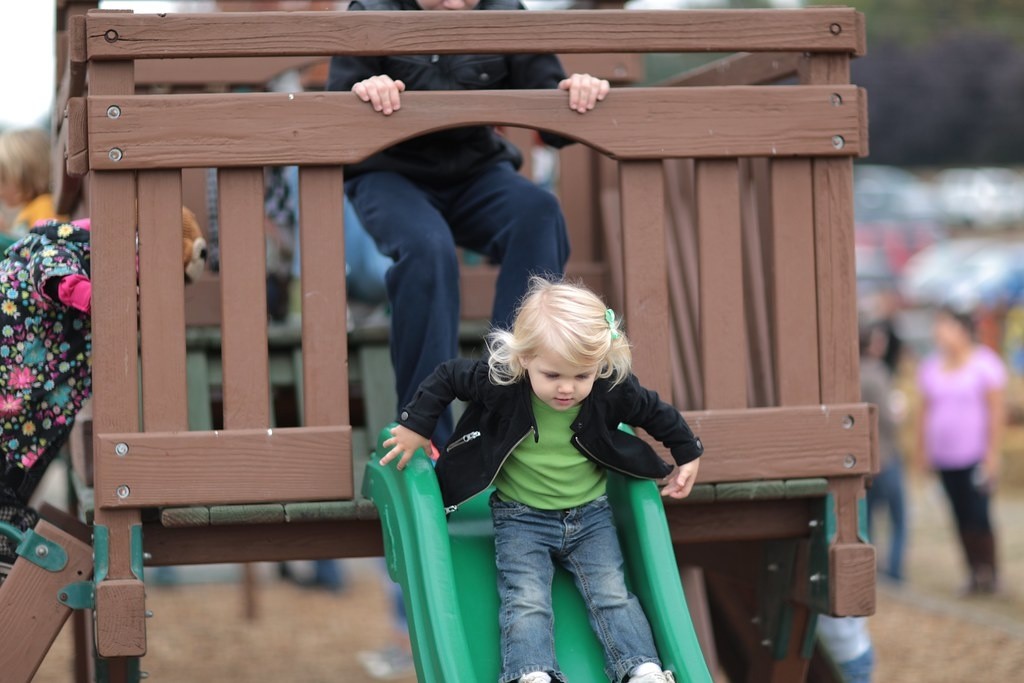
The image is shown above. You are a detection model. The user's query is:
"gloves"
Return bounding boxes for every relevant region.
[971,464,995,492]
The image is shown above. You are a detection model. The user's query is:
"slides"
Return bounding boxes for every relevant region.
[361,422,714,683]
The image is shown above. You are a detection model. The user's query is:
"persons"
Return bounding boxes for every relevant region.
[377,284,700,683]
[0,203,206,589]
[329,0,611,456]
[855,321,906,583]
[916,299,1009,597]
[207,70,394,587]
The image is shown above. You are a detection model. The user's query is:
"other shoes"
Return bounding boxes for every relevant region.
[628,670,675,683]
